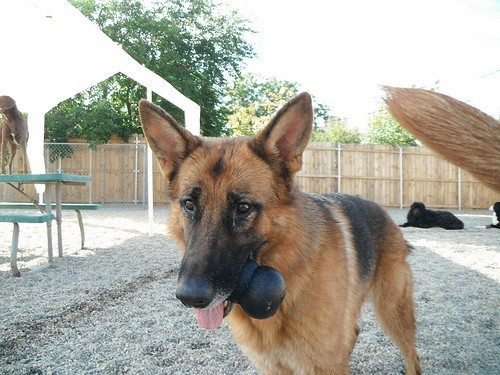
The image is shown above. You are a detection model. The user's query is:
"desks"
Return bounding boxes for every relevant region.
[0,174,94,262]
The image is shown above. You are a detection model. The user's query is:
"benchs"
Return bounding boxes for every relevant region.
[0,203,99,277]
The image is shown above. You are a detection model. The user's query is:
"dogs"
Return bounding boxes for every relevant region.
[398,202,464,230]
[0,95,29,175]
[475,202,500,229]
[376,83,500,194]
[138,91,422,375]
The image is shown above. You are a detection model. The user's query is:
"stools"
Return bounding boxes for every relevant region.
[0,210,54,279]
